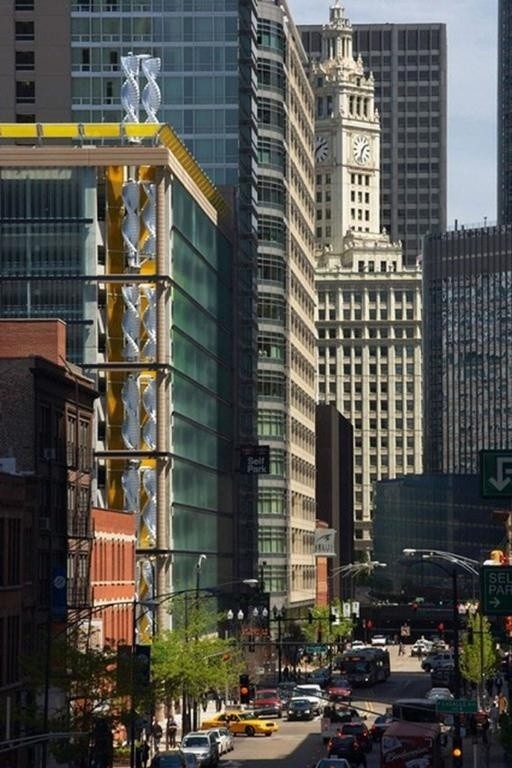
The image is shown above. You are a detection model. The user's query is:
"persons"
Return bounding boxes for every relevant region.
[481,714,491,745]
[138,738,152,767]
[397,639,404,657]
[223,713,234,733]
[469,713,478,745]
[416,640,423,662]
[166,714,180,749]
[401,646,406,657]
[150,717,164,756]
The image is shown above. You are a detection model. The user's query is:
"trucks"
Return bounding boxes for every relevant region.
[381,720,449,767]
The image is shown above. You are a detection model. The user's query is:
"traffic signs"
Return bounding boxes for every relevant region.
[480,565,512,614]
[305,647,326,653]
[436,700,477,713]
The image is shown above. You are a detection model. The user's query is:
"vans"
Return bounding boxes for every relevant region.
[292,683,324,714]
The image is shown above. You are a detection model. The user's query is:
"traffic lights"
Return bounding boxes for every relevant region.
[414,602,417,613]
[239,674,249,703]
[453,737,463,764]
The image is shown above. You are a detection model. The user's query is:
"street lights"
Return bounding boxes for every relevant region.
[252,605,269,629]
[226,608,245,642]
[131,576,260,768]
[457,600,478,646]
[403,546,485,688]
[41,599,159,768]
[273,605,287,681]
[399,558,459,639]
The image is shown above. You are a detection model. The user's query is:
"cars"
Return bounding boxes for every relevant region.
[278,680,298,707]
[352,639,366,649]
[287,699,314,720]
[315,706,394,768]
[201,710,279,736]
[371,633,387,645]
[149,728,234,768]
[309,668,330,688]
[412,637,456,701]
[325,679,353,700]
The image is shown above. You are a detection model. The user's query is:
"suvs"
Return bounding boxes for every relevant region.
[254,688,282,719]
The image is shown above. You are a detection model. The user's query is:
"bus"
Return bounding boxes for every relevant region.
[339,646,390,686]
